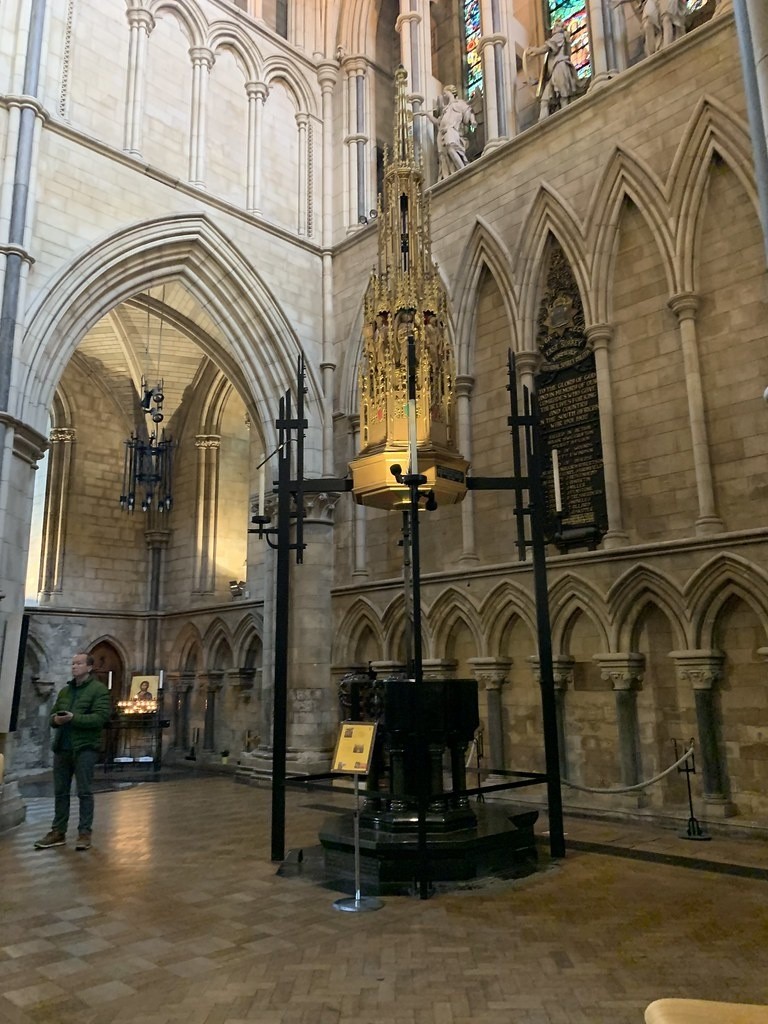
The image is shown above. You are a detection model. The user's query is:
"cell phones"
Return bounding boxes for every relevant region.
[56,711,67,717]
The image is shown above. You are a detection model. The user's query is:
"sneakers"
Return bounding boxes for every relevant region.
[76,833,92,849]
[34,831,66,847]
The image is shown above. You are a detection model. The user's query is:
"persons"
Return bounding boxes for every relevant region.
[34,653,113,850]
[136,681,153,699]
[421,85,477,180]
[526,21,578,121]
[642,0,686,56]
